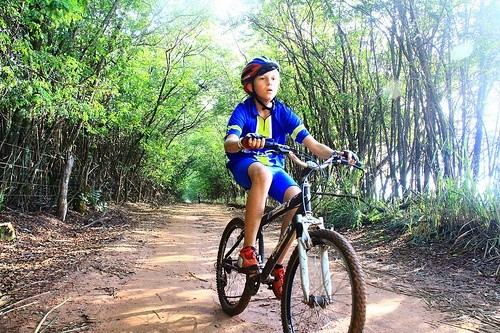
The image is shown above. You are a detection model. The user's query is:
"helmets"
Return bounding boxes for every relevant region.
[241,56,280,85]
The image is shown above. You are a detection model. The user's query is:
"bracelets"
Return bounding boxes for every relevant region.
[332,151,339,154]
[238,137,245,149]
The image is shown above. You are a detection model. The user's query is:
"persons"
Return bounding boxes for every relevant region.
[198,192,201,202]
[224,56,359,299]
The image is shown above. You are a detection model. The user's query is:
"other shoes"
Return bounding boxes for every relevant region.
[236,246,259,272]
[271,266,285,298]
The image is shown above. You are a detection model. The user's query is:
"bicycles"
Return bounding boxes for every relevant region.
[213,134,367,333]
